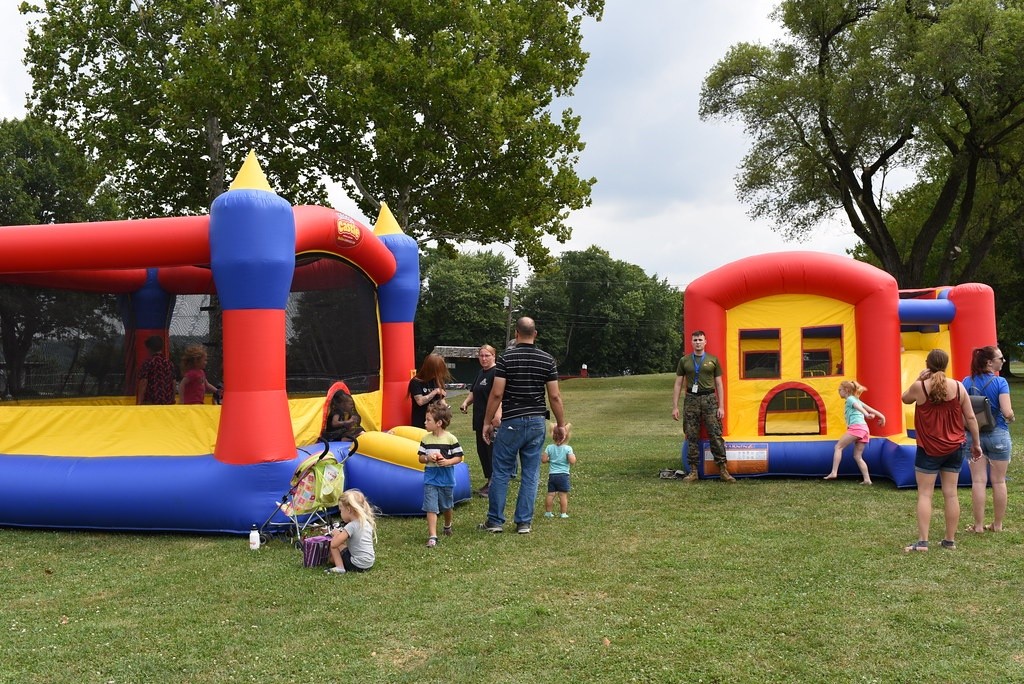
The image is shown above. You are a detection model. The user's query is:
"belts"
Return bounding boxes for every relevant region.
[687,388,715,396]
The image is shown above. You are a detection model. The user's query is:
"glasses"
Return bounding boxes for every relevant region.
[478,355,491,357]
[993,356,1003,363]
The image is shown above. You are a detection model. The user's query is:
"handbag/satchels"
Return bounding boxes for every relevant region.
[311,463,345,509]
[303,536,332,567]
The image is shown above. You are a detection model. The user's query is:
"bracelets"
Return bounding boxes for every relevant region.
[425,455,428,462]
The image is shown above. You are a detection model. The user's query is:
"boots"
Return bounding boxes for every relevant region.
[720,463,736,483]
[683,464,698,481]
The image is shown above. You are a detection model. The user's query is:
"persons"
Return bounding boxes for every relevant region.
[325,390,367,441]
[417,404,465,548]
[136,335,177,405]
[179,343,224,405]
[407,353,450,430]
[901,348,983,552]
[460,315,578,535]
[324,488,377,575]
[962,345,1015,533]
[671,329,736,483]
[823,381,886,485]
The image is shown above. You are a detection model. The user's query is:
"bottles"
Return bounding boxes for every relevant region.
[250,524,260,550]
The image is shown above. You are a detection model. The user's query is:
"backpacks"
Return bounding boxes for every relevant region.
[962,376,1000,432]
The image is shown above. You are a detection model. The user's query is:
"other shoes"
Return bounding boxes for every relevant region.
[905,541,928,551]
[560,514,569,518]
[444,525,452,536]
[544,512,555,518]
[477,522,503,533]
[983,522,1002,532]
[324,567,346,574]
[516,523,531,534]
[426,537,438,546]
[964,526,984,534]
[937,539,956,550]
[473,483,491,498]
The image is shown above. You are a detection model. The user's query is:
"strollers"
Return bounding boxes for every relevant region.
[258,433,359,552]
